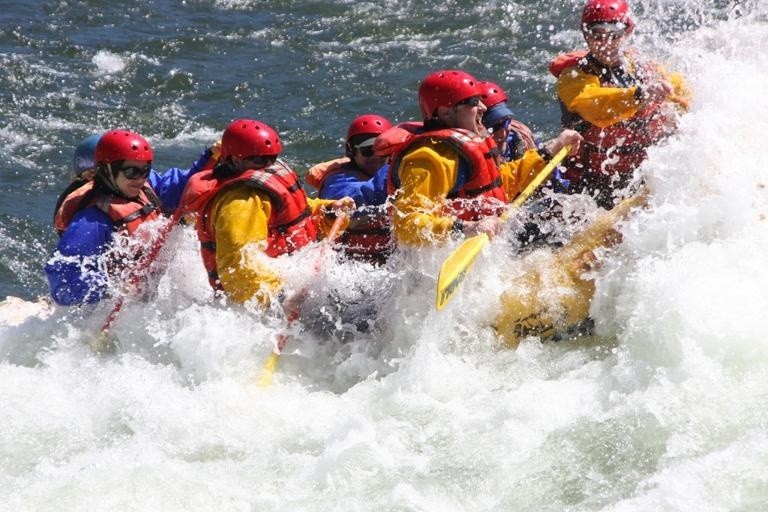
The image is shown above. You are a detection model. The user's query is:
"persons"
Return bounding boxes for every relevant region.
[546,0,696,212]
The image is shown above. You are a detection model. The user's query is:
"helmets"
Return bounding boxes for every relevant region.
[416,67,492,122]
[342,113,395,143]
[579,0,634,27]
[72,133,107,179]
[94,128,155,169]
[474,78,510,114]
[218,115,285,163]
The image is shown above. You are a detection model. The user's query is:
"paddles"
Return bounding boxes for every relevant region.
[92,152,219,351]
[259,212,345,388]
[436,145,573,308]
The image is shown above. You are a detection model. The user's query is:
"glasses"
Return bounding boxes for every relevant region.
[112,163,153,182]
[486,117,514,132]
[582,25,628,43]
[352,144,375,157]
[246,155,278,165]
[454,95,483,108]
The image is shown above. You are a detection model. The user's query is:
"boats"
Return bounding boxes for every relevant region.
[485,186,658,359]
